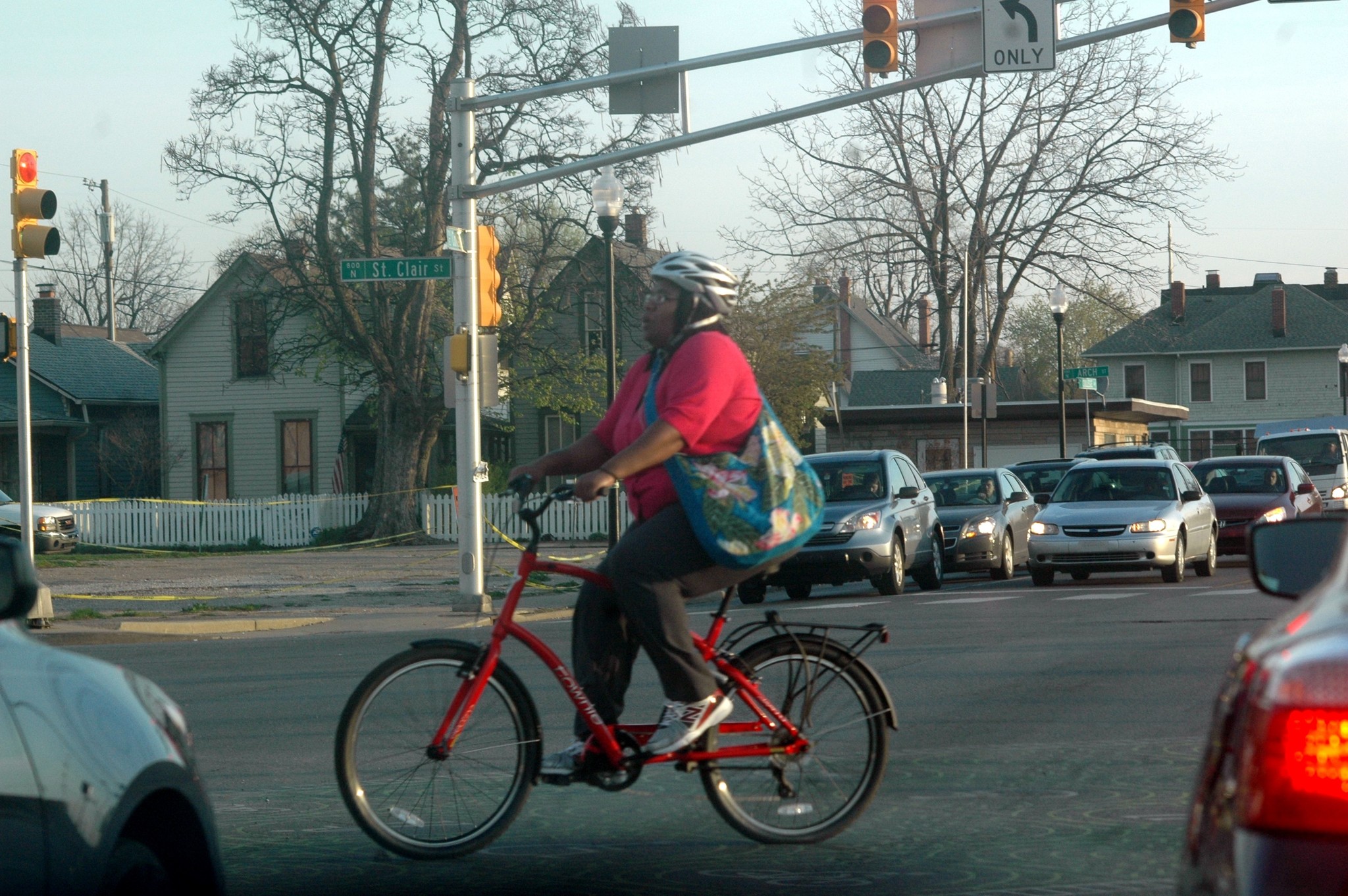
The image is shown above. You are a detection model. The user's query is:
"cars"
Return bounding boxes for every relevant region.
[918,469,1038,580]
[1026,459,1222,589]
[1184,453,1324,561]
[1004,455,1094,519]
[736,447,952,594]
[1168,503,1348,896]
[1,529,227,896]
[0,488,78,554]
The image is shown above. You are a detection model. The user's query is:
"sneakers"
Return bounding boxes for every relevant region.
[646,687,733,756]
[538,740,589,775]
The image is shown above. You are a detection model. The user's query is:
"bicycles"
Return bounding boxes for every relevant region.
[329,465,904,851]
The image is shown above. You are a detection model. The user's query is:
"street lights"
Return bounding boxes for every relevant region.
[1050,283,1070,458]
[592,160,620,556]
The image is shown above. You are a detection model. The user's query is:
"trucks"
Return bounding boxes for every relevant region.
[1256,428,1348,516]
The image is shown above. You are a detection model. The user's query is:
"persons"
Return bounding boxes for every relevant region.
[863,472,883,498]
[976,477,996,504]
[1317,440,1340,463]
[1264,468,1277,487]
[508,249,803,771]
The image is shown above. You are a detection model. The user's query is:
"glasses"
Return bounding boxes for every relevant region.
[642,291,679,304]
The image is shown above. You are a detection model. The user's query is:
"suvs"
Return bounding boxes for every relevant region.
[1072,444,1184,472]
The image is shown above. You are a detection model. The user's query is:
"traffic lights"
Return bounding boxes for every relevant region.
[10,148,60,259]
[862,0,898,73]
[1169,0,1204,44]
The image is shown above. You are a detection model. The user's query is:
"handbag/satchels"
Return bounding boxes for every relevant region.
[641,336,825,568]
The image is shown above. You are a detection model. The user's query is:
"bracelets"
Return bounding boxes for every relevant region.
[598,468,622,483]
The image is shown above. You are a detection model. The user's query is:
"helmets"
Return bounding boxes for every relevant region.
[650,250,743,318]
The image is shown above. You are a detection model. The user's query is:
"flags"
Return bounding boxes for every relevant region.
[332,425,351,495]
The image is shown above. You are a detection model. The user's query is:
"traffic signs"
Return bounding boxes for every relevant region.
[980,0,1058,71]
